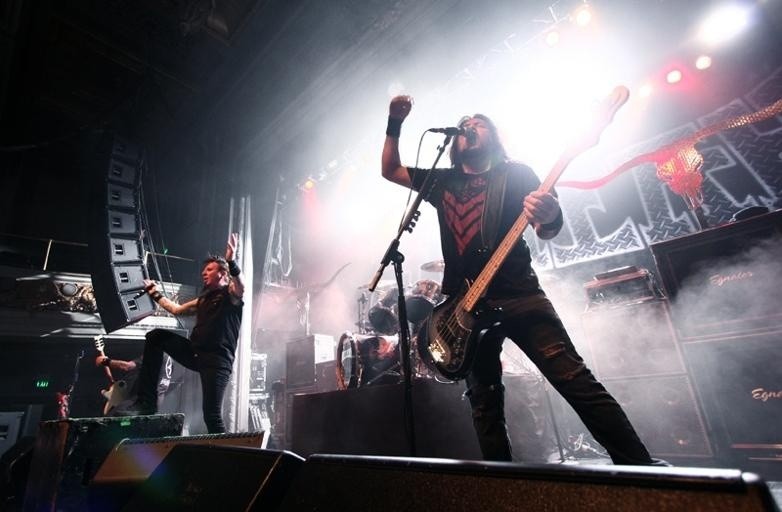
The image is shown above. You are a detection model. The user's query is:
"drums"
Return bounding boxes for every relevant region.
[406,279,445,325]
[369,287,409,337]
[336,332,395,391]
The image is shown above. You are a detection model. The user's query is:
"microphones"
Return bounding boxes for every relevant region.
[428,126,474,138]
[134,280,160,299]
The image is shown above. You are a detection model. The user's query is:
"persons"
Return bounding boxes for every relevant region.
[382,94,652,465]
[56,392,70,417]
[95,352,172,414]
[116,232,245,434]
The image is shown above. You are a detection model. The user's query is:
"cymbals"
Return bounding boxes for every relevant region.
[420,260,445,273]
[358,279,396,289]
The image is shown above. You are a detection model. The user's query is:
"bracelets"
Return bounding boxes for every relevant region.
[150,291,164,303]
[104,358,111,366]
[228,261,240,276]
[386,116,402,137]
[542,208,563,230]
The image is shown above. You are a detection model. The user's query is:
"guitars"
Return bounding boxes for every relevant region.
[417,84,629,378]
[94,333,128,415]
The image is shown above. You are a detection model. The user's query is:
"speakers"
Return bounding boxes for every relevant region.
[581,268,665,308]
[648,206,782,344]
[87,139,153,333]
[685,328,782,460]
[284,391,324,453]
[286,334,336,391]
[597,372,730,460]
[119,443,307,512]
[288,453,779,512]
[580,303,696,382]
